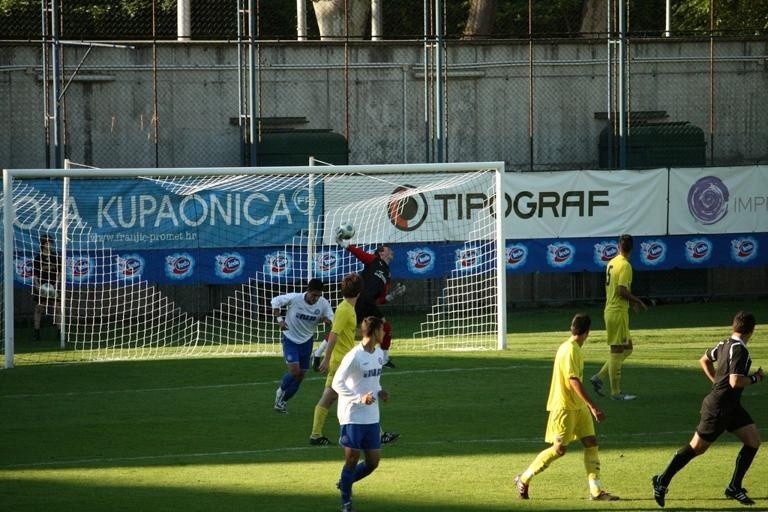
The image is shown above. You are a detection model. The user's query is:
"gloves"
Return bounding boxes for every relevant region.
[385,281,407,303]
[334,235,350,250]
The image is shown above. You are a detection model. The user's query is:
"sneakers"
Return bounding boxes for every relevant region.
[380,431,400,444]
[724,482,756,506]
[312,350,322,372]
[589,374,606,397]
[514,473,529,500]
[379,359,396,370]
[309,435,331,446]
[589,490,620,502]
[651,474,666,507]
[273,384,290,417]
[609,391,638,403]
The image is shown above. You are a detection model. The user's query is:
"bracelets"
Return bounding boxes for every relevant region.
[275,316,283,322]
[749,375,757,383]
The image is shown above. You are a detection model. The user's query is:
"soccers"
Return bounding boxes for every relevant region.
[41,284,54,297]
[338,221,356,240]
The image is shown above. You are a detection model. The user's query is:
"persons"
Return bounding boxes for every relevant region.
[271,278,335,413]
[31,234,69,341]
[514,312,620,501]
[312,225,406,372]
[309,273,402,446]
[330,315,389,511]
[648,305,766,506]
[587,234,648,401]
